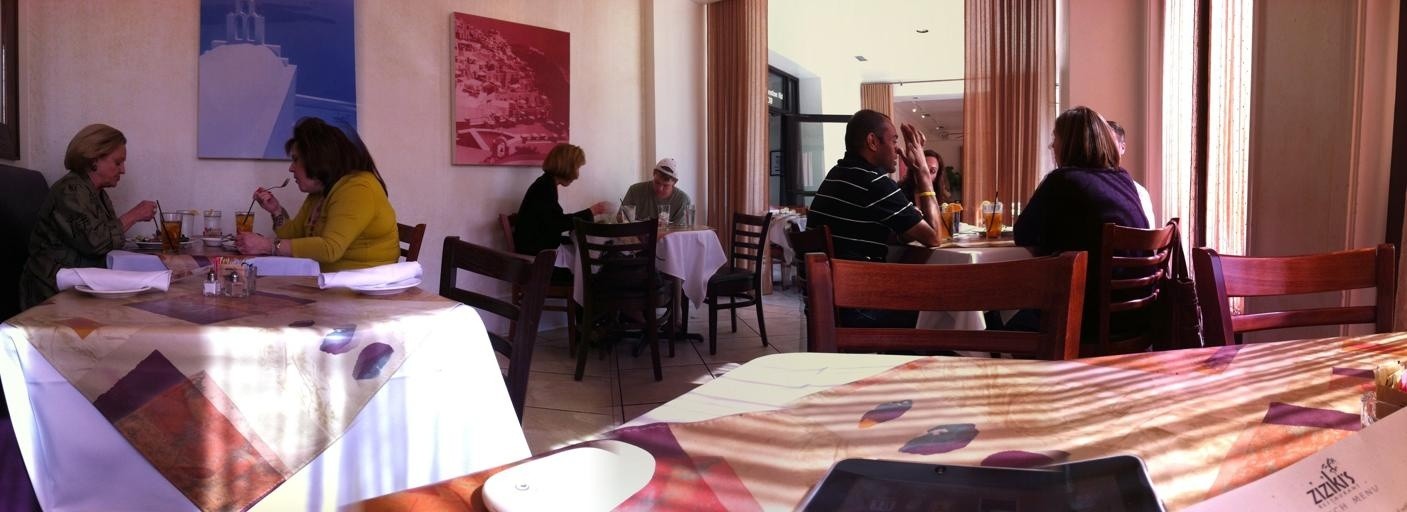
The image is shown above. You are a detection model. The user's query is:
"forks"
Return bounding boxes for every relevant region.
[261,178,290,190]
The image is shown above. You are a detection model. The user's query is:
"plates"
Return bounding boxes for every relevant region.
[222,240,238,252]
[350,278,421,295]
[73,284,151,299]
[135,240,196,249]
[201,238,228,248]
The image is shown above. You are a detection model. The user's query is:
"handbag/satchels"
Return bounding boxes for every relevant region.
[1157,220,1202,350]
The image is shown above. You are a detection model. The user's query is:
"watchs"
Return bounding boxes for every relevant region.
[272,238,282,258]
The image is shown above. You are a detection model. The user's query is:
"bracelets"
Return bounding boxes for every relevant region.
[270,207,289,228]
[916,190,936,201]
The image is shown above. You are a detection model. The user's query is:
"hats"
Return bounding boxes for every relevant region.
[652,157,679,180]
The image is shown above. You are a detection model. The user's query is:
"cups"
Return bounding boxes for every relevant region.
[178,209,194,238]
[950,203,961,233]
[160,212,183,252]
[621,206,635,224]
[237,211,254,235]
[982,203,1002,238]
[657,205,670,225]
[939,205,953,241]
[204,210,221,238]
[685,204,695,226]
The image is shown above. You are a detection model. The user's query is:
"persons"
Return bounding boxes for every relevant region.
[896,150,953,223]
[1007,105,1156,358]
[1109,120,1154,228]
[615,158,690,227]
[803,109,961,356]
[514,142,617,331]
[233,117,401,273]
[8,124,157,316]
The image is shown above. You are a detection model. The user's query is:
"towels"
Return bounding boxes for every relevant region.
[55,268,173,293]
[318,260,422,289]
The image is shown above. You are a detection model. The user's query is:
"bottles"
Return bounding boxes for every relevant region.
[225,271,244,298]
[202,271,221,296]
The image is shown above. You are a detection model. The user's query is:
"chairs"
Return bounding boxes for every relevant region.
[680,211,771,355]
[571,215,663,383]
[801,251,1090,362]
[786,224,884,352]
[439,236,558,429]
[499,211,583,356]
[395,223,428,262]
[1,165,49,321]
[990,217,1183,358]
[1193,244,1395,346]
